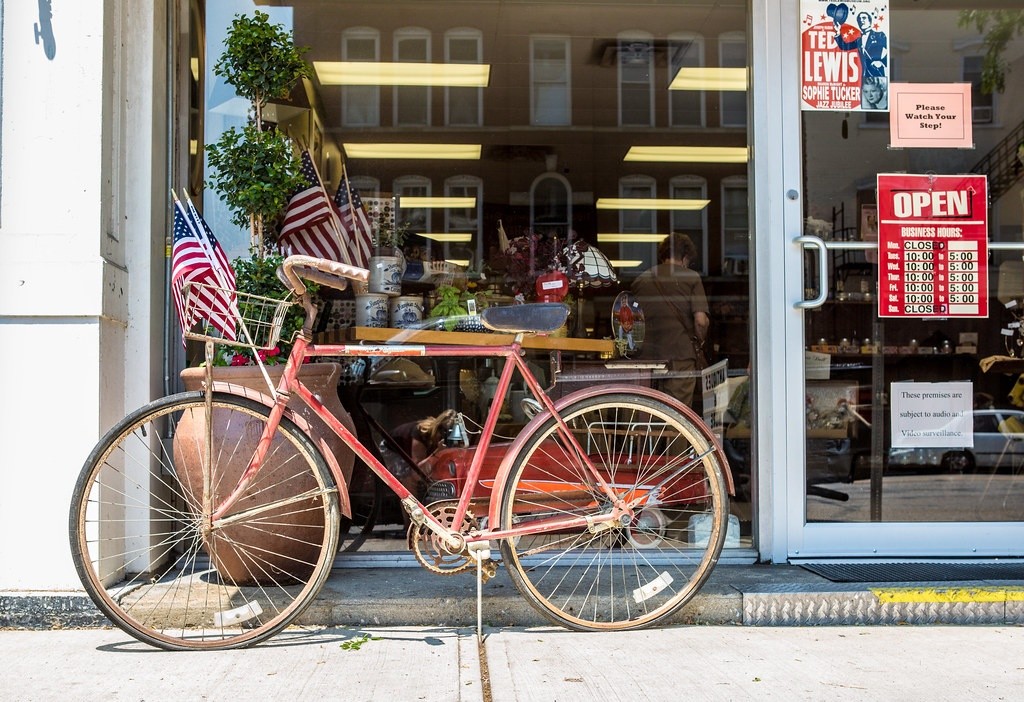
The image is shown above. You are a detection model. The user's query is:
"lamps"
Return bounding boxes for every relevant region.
[621,44,649,83]
[549,238,617,338]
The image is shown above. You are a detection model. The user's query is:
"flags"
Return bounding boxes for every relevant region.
[172,199,238,352]
[278,150,373,279]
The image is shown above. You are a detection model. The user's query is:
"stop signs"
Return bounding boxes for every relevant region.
[536,270,570,301]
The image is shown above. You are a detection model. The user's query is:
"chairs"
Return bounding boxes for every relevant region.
[977,404,1024,517]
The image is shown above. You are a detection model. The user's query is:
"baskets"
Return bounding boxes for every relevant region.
[181,282,293,351]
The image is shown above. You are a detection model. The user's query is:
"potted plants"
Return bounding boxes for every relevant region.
[373,216,409,256]
[173,10,357,587]
[431,285,494,334]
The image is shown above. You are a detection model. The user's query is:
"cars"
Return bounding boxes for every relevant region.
[891,408,1024,474]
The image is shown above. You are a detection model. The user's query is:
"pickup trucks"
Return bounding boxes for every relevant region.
[338,312,852,503]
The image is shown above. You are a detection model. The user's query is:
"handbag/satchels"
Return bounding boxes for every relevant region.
[695,348,708,371]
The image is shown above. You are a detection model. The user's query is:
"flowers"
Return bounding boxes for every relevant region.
[193,345,322,366]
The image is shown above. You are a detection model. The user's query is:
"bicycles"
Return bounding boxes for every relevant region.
[67,255,735,651]
[335,338,441,551]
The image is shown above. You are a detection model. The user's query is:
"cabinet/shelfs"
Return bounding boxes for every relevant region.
[702,278,984,476]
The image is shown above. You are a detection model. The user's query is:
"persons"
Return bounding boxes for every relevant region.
[619,295,635,350]
[378,409,465,538]
[631,234,709,456]
[861,77,887,108]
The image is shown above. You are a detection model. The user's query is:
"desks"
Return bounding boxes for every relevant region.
[313,330,613,403]
